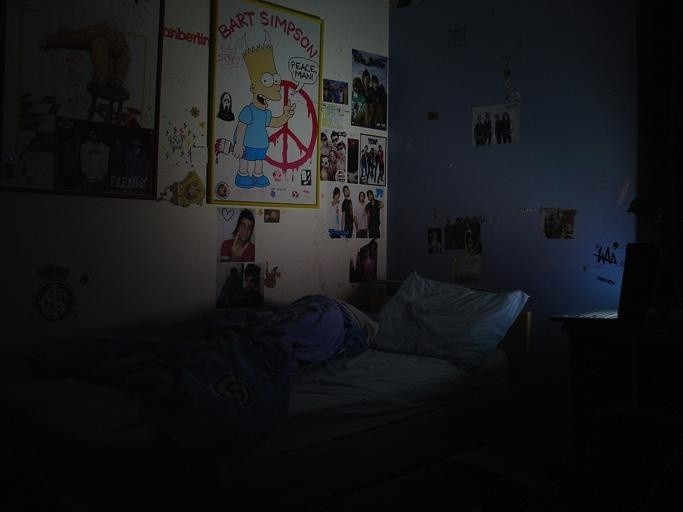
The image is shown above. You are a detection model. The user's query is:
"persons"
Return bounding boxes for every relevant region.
[321,130,384,238]
[221,210,253,262]
[236,265,262,307]
[352,69,386,130]
[475,111,513,144]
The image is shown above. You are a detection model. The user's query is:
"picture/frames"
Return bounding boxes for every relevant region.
[201,0,325,211]
[0,2,167,208]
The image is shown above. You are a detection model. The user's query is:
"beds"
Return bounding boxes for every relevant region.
[0,277,544,509]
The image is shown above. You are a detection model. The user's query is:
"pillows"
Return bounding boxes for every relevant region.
[358,269,533,373]
